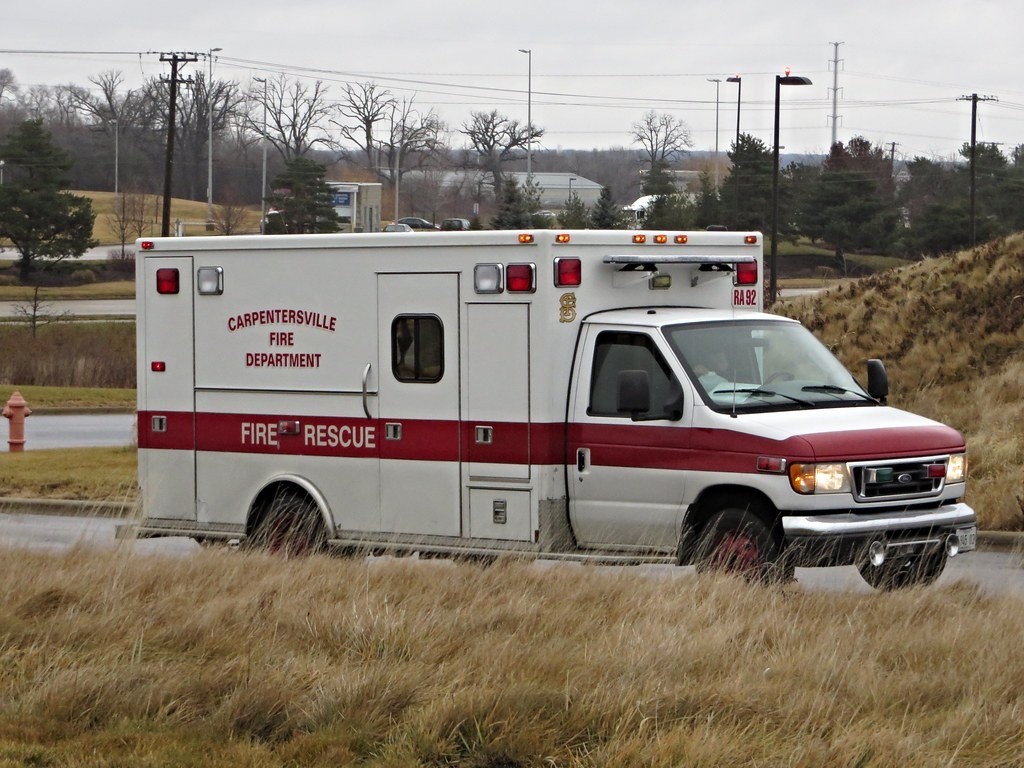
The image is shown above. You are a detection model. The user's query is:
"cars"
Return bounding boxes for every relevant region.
[382,223,414,232]
[532,209,556,217]
[397,217,439,231]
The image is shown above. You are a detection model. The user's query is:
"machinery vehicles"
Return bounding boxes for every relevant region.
[619,192,697,229]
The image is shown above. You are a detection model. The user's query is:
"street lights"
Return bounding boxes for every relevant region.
[769,68,813,304]
[206,47,223,231]
[725,75,741,228]
[107,119,118,214]
[519,48,532,185]
[251,76,267,235]
[568,177,577,202]
[707,79,722,192]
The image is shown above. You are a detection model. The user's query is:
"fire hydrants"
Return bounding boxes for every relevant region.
[2,389,33,452]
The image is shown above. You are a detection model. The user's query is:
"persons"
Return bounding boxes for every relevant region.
[692,345,752,384]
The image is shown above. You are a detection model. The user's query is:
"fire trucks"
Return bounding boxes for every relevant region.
[115,229,976,593]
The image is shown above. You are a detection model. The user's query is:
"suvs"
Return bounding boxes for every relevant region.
[441,218,469,231]
